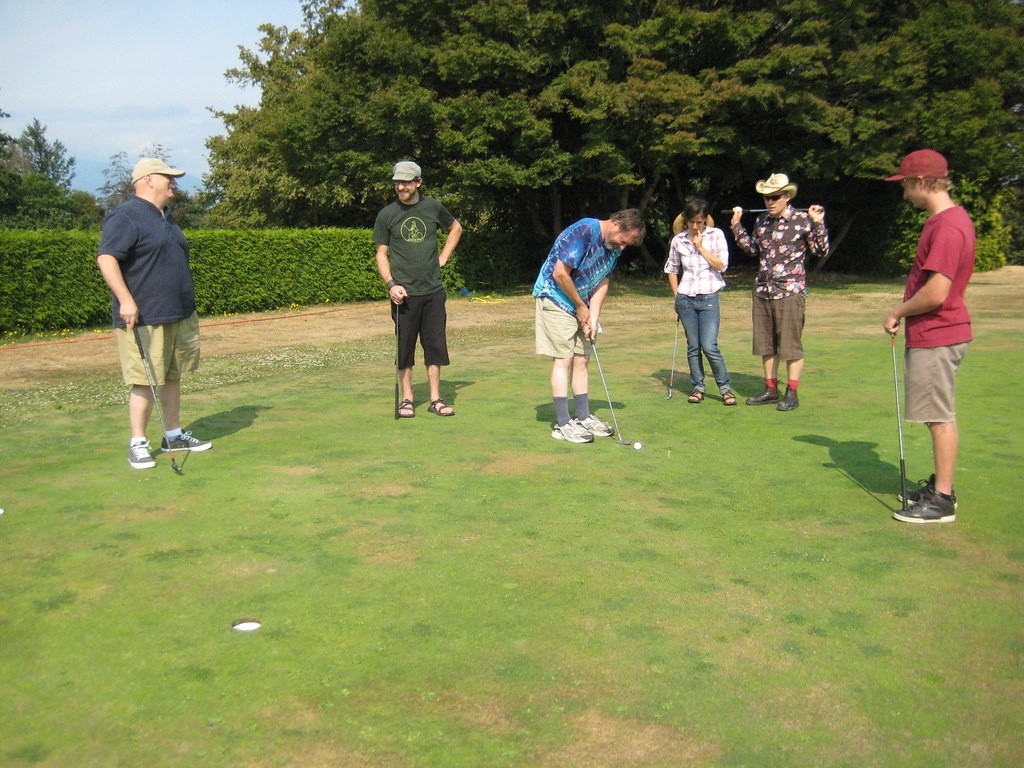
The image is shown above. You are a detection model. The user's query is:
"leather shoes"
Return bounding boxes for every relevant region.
[778,385,799,411]
[746,381,781,405]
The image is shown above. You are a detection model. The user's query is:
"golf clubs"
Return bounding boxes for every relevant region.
[892,321,907,512]
[719,207,824,214]
[394,290,403,419]
[589,336,632,445]
[666,313,681,400]
[133,323,182,472]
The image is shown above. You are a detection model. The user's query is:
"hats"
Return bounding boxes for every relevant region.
[884,150,948,181]
[756,173,797,199]
[132,158,186,185]
[392,161,421,181]
[673,212,714,235]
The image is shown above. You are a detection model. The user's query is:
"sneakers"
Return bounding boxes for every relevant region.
[163,430,213,452]
[573,414,613,437]
[551,419,594,443]
[893,488,955,524]
[898,474,958,510]
[129,439,156,469]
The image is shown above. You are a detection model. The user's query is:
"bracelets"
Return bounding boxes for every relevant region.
[386,280,395,291]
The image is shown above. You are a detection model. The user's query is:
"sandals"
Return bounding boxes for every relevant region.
[399,399,414,418]
[723,391,736,405]
[688,389,704,403]
[428,398,455,416]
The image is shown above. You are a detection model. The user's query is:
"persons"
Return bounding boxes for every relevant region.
[96,158,212,469]
[730,173,830,411]
[372,161,462,419]
[664,199,737,406]
[532,207,645,443]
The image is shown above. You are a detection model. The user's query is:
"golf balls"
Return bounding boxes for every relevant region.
[634,442,642,450]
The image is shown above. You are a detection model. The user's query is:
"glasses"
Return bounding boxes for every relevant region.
[394,180,417,186]
[766,192,788,201]
